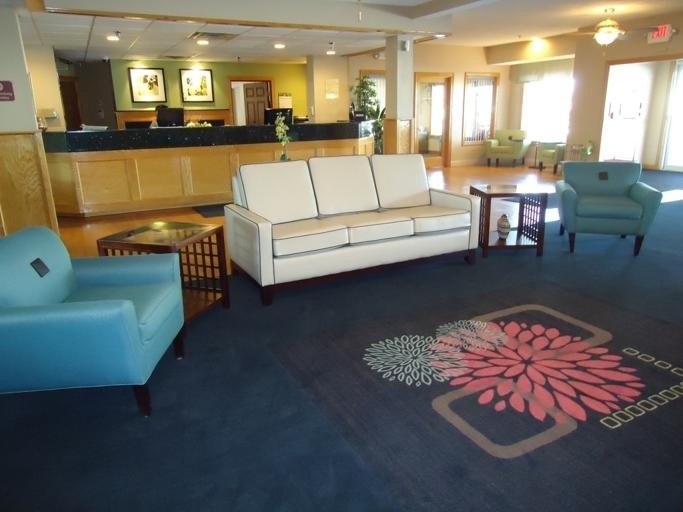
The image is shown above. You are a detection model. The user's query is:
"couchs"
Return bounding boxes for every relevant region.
[223,152,481,307]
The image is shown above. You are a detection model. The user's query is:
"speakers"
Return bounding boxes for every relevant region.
[402,40,409,51]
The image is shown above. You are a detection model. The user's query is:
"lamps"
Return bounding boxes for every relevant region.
[590,27,619,48]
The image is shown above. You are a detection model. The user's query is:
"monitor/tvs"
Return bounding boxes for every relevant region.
[158,107,184,126]
[264,107,293,125]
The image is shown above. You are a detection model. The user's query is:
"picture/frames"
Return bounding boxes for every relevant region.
[127,65,215,103]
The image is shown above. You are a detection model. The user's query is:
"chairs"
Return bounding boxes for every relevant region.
[0,224,184,418]
[485,129,566,174]
[555,161,664,256]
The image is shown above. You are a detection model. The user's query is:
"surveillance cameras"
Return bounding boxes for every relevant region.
[326,49,335,56]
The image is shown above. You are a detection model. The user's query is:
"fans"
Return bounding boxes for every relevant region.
[566,8,660,41]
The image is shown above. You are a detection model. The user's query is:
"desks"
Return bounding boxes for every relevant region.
[468,183,548,258]
[95,221,231,330]
